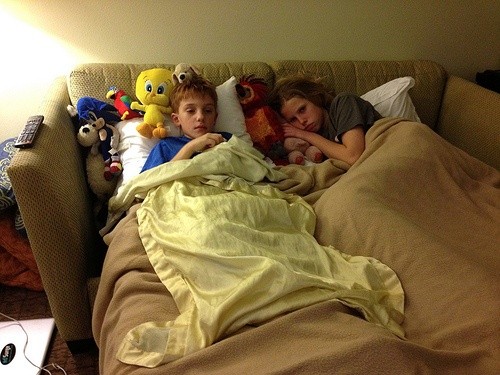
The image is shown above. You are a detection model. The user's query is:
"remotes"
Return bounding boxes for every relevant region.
[15,115,44,148]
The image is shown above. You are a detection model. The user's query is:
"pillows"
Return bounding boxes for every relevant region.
[359,76,422,124]
[110,76,254,202]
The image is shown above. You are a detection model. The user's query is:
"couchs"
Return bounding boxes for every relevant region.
[7,61,500,355]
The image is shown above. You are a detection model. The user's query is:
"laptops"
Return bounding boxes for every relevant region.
[0,318,55,375]
